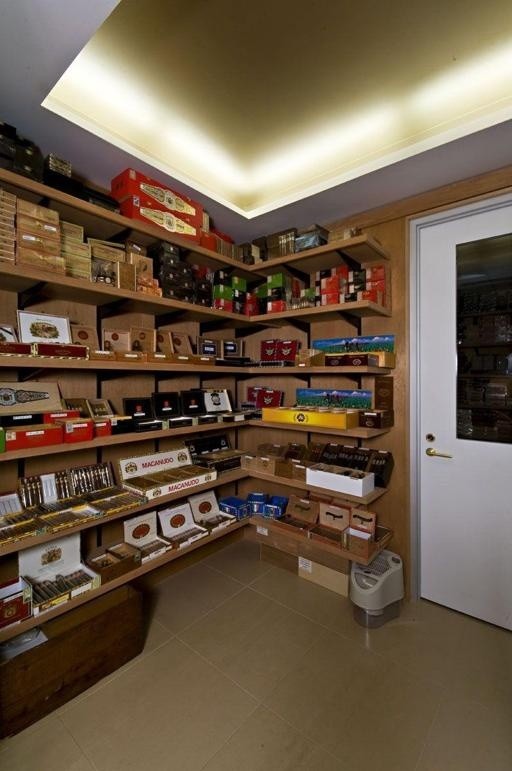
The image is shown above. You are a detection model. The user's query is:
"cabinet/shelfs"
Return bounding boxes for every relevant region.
[0,167,394,644]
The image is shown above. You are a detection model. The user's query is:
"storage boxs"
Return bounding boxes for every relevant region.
[297,556,350,598]
[0,582,145,740]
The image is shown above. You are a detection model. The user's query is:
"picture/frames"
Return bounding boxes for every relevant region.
[0,309,244,359]
